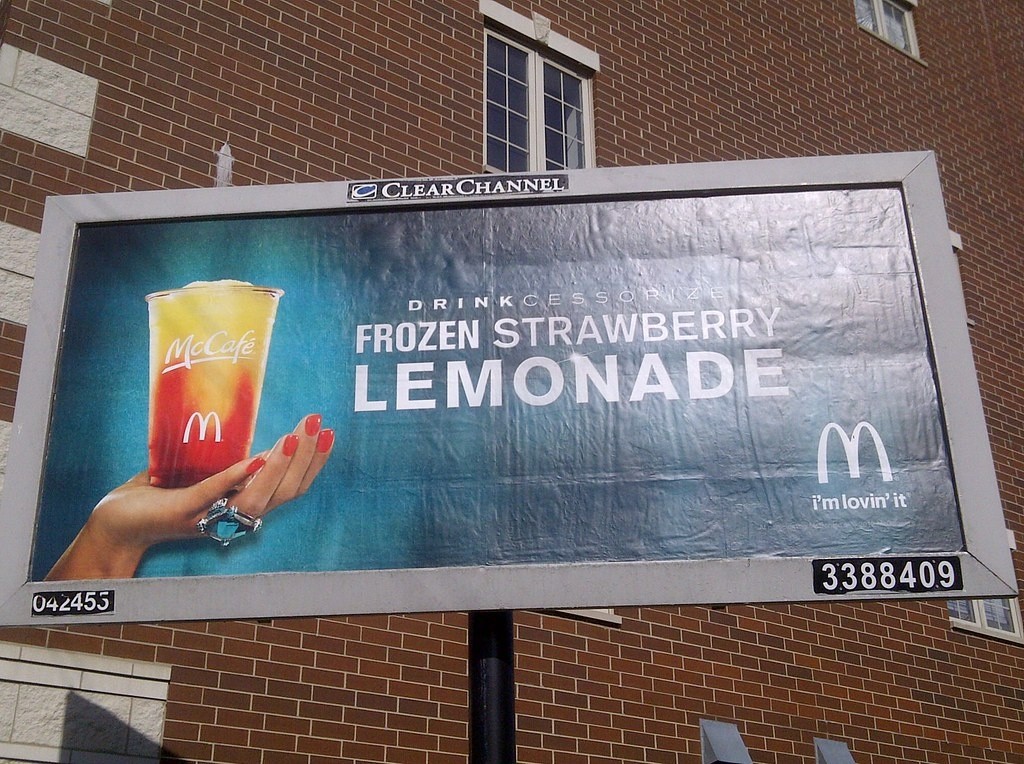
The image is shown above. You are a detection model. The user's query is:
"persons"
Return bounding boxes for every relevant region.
[44,414,335,583]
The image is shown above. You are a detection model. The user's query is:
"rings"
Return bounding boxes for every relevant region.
[196,498,263,547]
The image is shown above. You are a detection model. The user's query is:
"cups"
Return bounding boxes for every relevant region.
[145,285,285,488]
